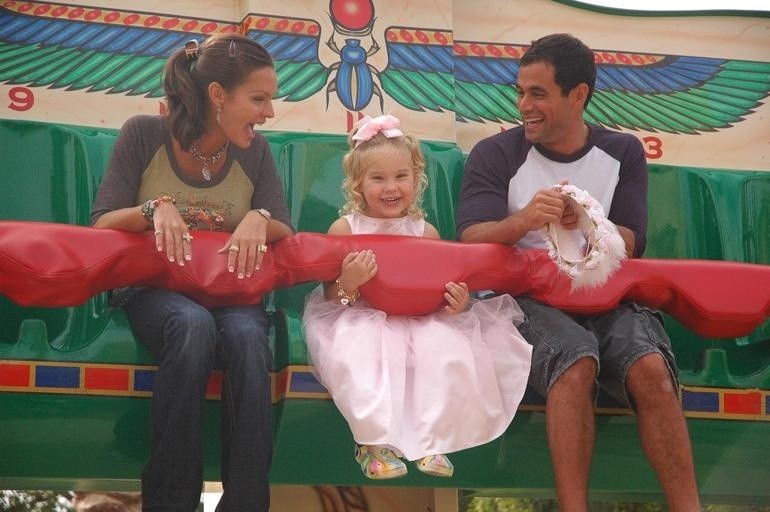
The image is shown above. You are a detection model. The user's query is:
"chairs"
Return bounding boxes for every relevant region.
[1,114,770,499]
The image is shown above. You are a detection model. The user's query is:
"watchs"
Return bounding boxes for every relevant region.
[252,208,272,225]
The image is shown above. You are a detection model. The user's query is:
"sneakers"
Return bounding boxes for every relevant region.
[354,444,408,480]
[415,455,455,478]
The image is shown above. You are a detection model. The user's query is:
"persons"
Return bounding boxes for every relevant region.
[300,116,533,481]
[90,34,296,511]
[454,32,704,512]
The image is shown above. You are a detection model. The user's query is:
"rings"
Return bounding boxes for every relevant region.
[228,246,240,253]
[182,233,194,242]
[154,231,164,236]
[257,244,267,254]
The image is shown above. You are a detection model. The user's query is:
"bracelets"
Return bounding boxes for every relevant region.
[141,195,176,224]
[335,276,361,306]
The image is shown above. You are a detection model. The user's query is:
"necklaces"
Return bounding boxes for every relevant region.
[188,136,230,182]
